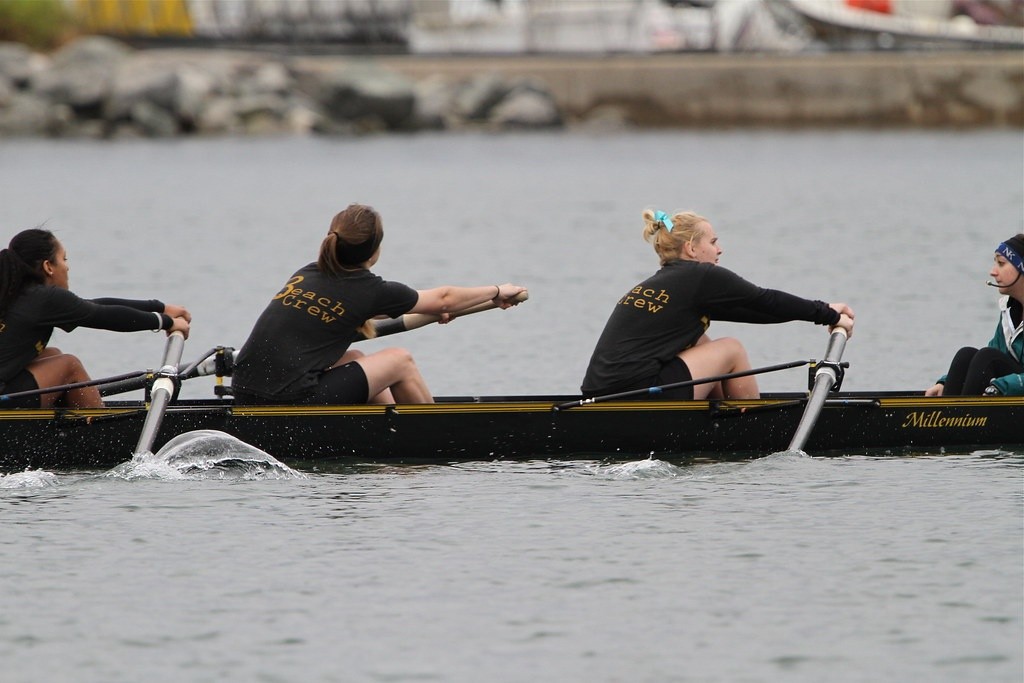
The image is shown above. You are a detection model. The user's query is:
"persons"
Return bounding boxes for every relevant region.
[925,234,1024,399]
[580,212,854,404]
[0,227,193,412]
[231,204,527,407]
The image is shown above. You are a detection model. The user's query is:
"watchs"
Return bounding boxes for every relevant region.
[983,386,998,396]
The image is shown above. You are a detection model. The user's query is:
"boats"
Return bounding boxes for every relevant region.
[0,391,1023,470]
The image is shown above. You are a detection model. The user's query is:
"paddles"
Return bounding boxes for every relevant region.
[130,315,188,463]
[787,312,849,453]
[94,289,531,397]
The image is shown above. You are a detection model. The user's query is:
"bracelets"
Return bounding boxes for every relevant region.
[152,311,162,333]
[491,285,499,299]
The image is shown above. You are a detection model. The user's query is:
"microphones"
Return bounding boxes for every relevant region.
[986,272,1021,288]
